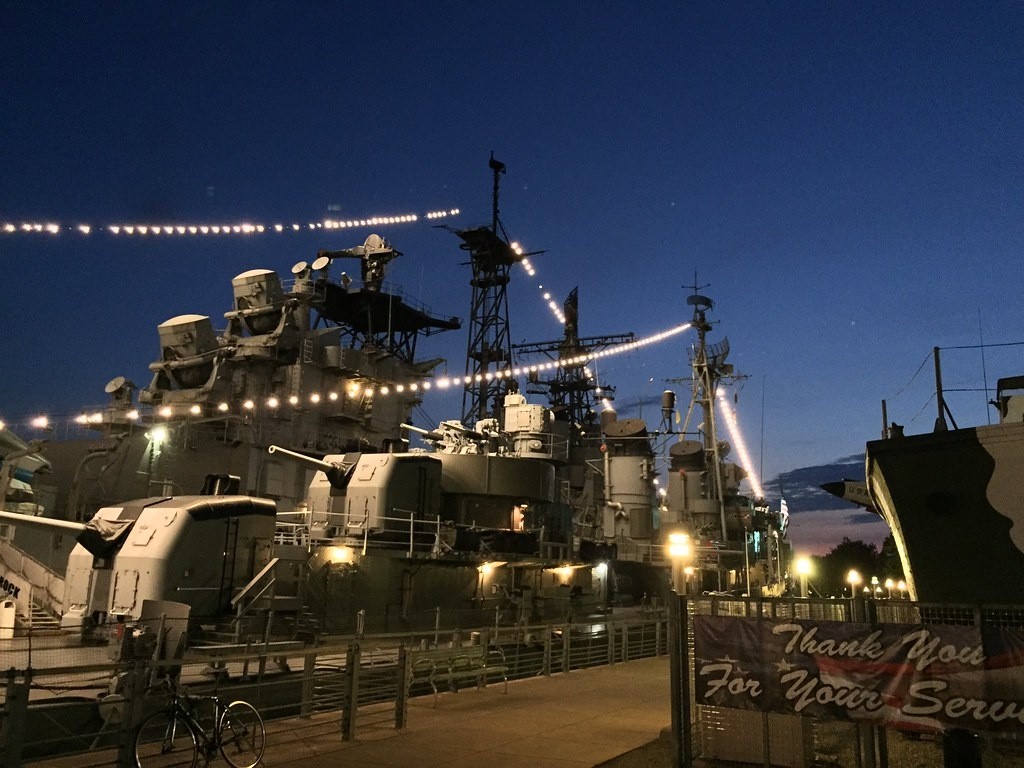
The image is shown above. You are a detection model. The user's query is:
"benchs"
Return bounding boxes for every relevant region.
[645,609,664,620]
[411,644,508,709]
[189,641,303,668]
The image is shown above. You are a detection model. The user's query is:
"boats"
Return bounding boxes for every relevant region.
[0,150,1024,768]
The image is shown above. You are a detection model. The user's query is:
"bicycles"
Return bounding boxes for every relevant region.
[126,661,268,767]
